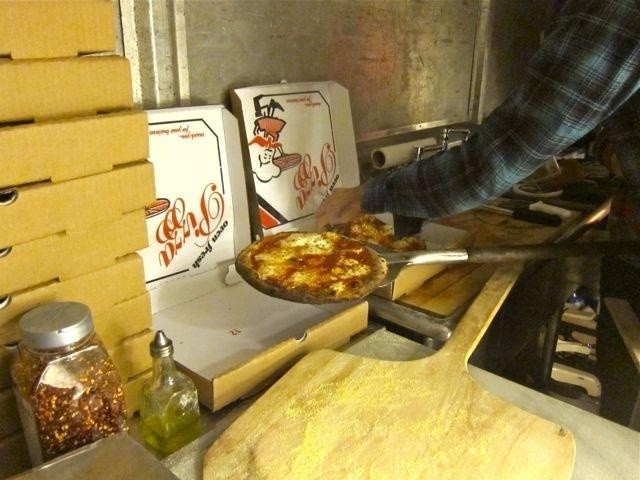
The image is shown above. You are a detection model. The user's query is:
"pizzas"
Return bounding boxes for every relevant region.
[320,217,428,255]
[232,230,391,306]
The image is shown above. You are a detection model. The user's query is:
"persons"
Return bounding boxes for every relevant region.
[314,1,640,430]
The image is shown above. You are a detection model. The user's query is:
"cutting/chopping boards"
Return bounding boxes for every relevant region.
[197,246,576,480]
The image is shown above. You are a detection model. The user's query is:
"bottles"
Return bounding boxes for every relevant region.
[141,331,203,452]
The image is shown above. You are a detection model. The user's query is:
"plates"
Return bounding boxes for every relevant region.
[511,156,565,198]
